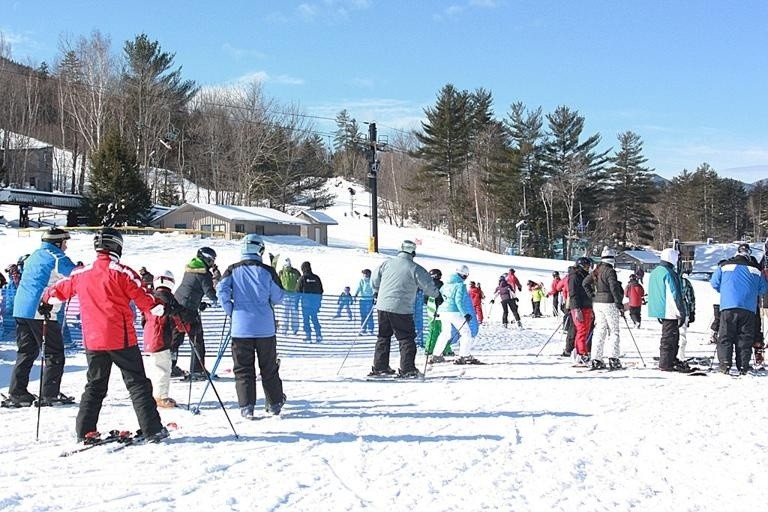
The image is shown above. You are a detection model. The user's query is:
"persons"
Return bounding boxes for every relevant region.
[3,226,73,407]
[215,232,287,418]
[0,244,321,406]
[42,228,182,448]
[336,237,768,376]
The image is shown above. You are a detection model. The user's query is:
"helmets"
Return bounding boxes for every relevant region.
[197,246,217,261]
[94,228,125,255]
[41,227,70,247]
[239,233,266,255]
[152,270,176,290]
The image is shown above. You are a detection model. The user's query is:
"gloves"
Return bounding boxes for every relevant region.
[689,314,696,323]
[198,302,206,311]
[657,317,663,325]
[436,291,443,306]
[464,314,473,321]
[677,318,685,328]
[167,304,179,316]
[575,310,584,321]
[37,303,52,314]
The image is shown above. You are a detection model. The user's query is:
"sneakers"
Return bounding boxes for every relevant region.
[172,366,216,381]
[269,393,287,415]
[156,397,176,409]
[400,368,424,379]
[561,350,623,369]
[504,313,542,331]
[241,408,254,419]
[302,335,322,345]
[459,356,475,364]
[11,394,66,404]
[651,357,766,376]
[372,366,396,376]
[146,427,170,445]
[429,355,445,362]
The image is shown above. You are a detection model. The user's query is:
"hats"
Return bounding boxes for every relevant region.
[498,268,516,281]
[401,239,416,254]
[552,270,559,279]
[576,257,592,267]
[601,245,615,260]
[455,264,470,276]
[660,246,679,267]
[301,261,312,273]
[429,269,442,279]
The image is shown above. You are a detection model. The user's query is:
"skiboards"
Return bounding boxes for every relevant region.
[60,423,177,456]
[170,372,234,381]
[1,400,80,407]
[342,374,459,381]
[573,364,611,371]
[426,359,494,365]
[714,364,768,376]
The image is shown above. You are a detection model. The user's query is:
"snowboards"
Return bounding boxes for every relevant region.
[652,360,707,377]
